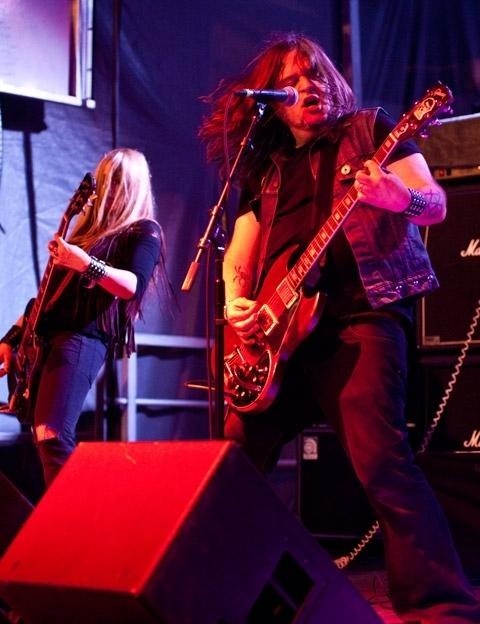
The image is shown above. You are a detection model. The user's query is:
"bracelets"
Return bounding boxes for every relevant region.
[222,298,233,322]
[1,325,22,347]
[83,256,105,281]
[403,188,427,216]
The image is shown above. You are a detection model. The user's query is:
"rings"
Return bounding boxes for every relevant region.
[53,247,57,256]
[358,184,363,192]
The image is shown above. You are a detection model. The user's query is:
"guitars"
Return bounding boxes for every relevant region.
[6,174,98,419]
[210,81,452,414]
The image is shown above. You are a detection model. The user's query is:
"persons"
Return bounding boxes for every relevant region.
[0,147,181,491]
[196,33,480,624]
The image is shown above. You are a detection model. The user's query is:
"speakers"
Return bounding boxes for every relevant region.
[403,353,480,452]
[297,428,385,563]
[1,439,384,624]
[410,180,479,353]
[412,454,479,587]
[1,470,34,554]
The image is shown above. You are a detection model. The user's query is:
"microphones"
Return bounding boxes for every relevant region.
[234,86,299,106]
